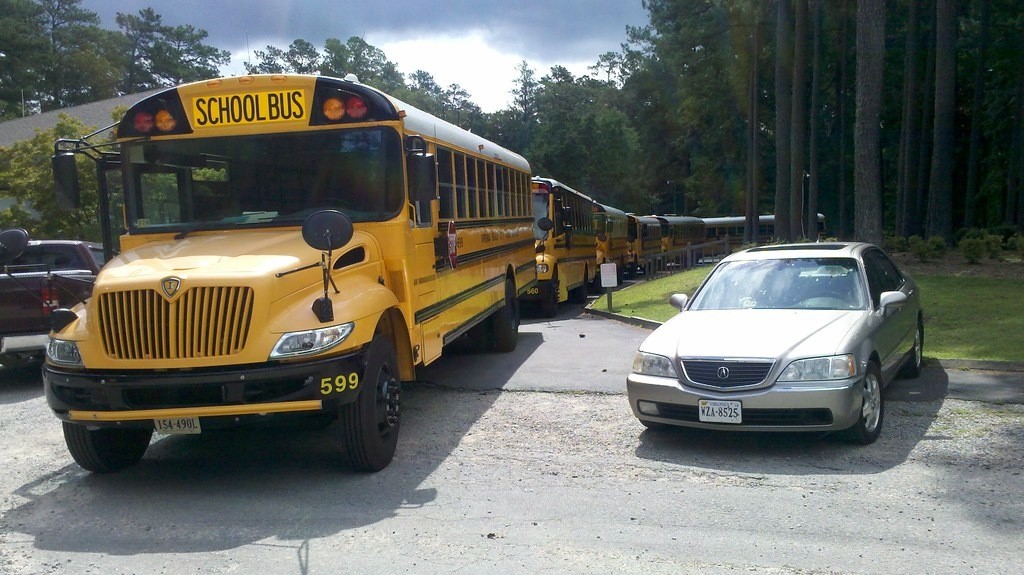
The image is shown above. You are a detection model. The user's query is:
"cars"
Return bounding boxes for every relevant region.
[625,243,924,446]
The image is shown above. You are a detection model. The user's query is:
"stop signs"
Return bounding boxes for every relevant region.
[447,221,459,268]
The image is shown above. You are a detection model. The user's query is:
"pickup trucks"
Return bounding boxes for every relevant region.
[0,241,120,354]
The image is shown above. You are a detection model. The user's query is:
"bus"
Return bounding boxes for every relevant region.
[703,217,775,255]
[43,73,540,478]
[519,177,597,316]
[593,203,630,285]
[627,214,662,274]
[657,217,705,264]
